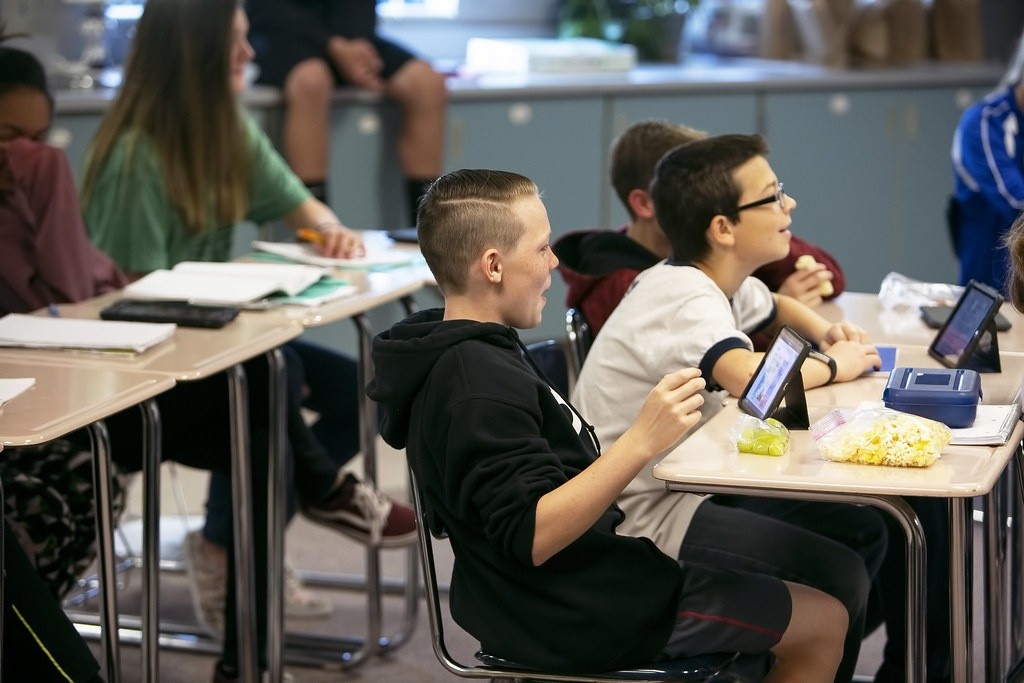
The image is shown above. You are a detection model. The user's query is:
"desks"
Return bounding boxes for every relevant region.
[1,228,436,682]
[652,284,1023,682]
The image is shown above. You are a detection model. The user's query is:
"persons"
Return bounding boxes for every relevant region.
[948,28,1024,295]
[80,1,419,633]
[365,169,851,683]
[0,51,335,683]
[568,131,881,683]
[549,121,955,683]
[244,0,447,223]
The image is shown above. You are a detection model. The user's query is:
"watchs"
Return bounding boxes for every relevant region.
[808,350,836,386]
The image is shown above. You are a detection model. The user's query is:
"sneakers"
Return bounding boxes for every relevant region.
[280,562,335,617]
[184,530,226,640]
[300,472,418,548]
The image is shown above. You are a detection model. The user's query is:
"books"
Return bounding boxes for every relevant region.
[943,403,1020,446]
[127,258,334,306]
[0,313,177,356]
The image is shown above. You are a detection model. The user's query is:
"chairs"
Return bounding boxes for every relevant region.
[406,303,747,683]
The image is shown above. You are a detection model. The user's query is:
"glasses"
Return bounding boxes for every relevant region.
[720,182,785,215]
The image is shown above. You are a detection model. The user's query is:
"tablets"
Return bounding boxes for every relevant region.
[926,280,1004,369]
[920,305,1013,331]
[101,298,239,329]
[386,227,418,243]
[738,324,812,421]
